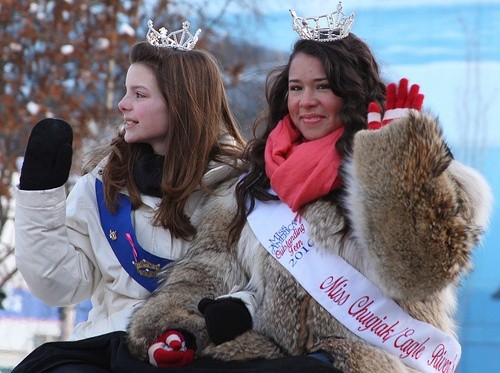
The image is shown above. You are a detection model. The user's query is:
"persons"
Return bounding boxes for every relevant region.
[127,27,493,372]
[10,40,258,373]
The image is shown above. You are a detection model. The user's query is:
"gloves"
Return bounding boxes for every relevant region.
[367,76,424,130]
[147,328,197,369]
[198,296,252,345]
[19,117,73,191]
